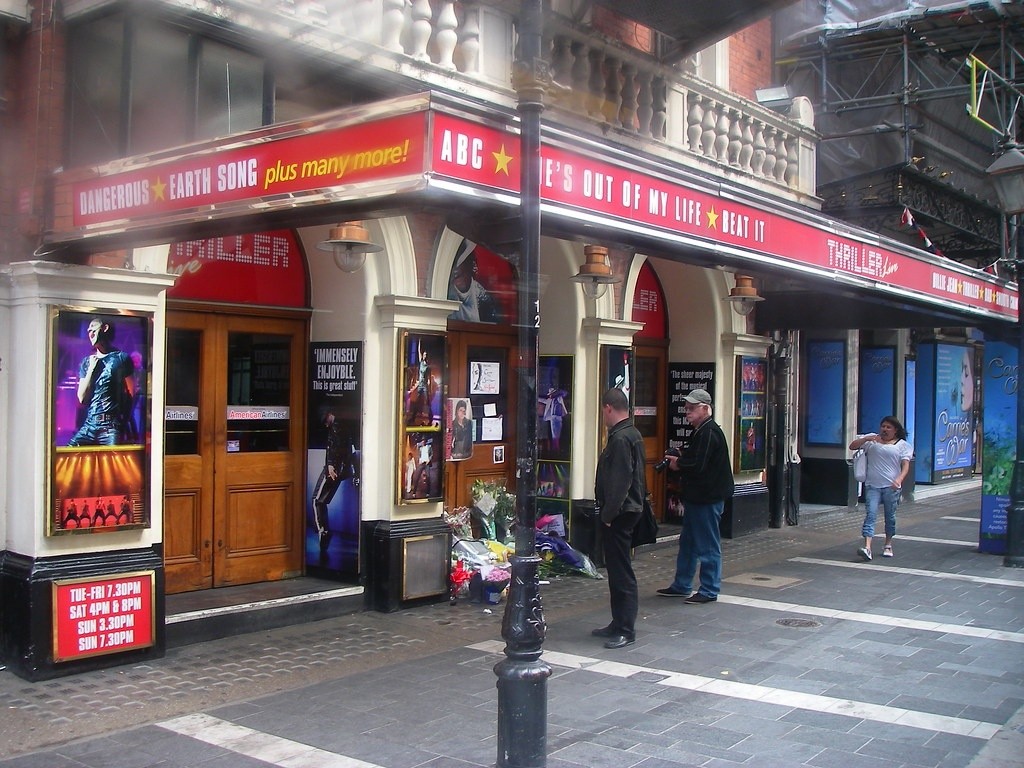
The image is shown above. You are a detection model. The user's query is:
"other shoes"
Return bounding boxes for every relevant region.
[882,544,893,556]
[857,547,872,562]
[683,593,717,604]
[319,530,333,555]
[656,587,690,596]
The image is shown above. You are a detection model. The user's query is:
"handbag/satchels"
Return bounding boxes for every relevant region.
[852,436,871,482]
[631,495,658,549]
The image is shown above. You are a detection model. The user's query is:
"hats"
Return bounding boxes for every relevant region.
[614,375,624,389]
[683,389,712,404]
[420,435,428,440]
[547,388,556,395]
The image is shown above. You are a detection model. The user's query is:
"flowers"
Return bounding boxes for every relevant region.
[442,478,604,607]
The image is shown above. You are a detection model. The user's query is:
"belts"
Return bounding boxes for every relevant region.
[92,413,115,421]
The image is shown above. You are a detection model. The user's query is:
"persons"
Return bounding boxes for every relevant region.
[62,495,134,529]
[405,438,434,499]
[959,346,976,427]
[65,315,138,448]
[451,399,473,459]
[591,388,647,649]
[537,388,568,451]
[849,416,911,562]
[312,408,359,555]
[743,360,764,455]
[406,334,434,427]
[449,253,496,322]
[614,351,630,414]
[655,389,735,604]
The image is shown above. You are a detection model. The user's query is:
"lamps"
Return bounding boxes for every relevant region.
[568,245,623,301]
[316,220,385,273]
[754,85,793,116]
[720,272,766,315]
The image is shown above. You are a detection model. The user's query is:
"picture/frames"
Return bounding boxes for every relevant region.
[597,344,637,463]
[392,326,449,506]
[733,354,768,474]
[42,304,154,539]
[538,354,576,541]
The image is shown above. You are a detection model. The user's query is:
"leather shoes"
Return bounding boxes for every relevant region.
[604,632,636,648]
[592,621,616,637]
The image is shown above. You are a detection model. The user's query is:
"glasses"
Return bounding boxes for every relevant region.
[684,404,705,412]
